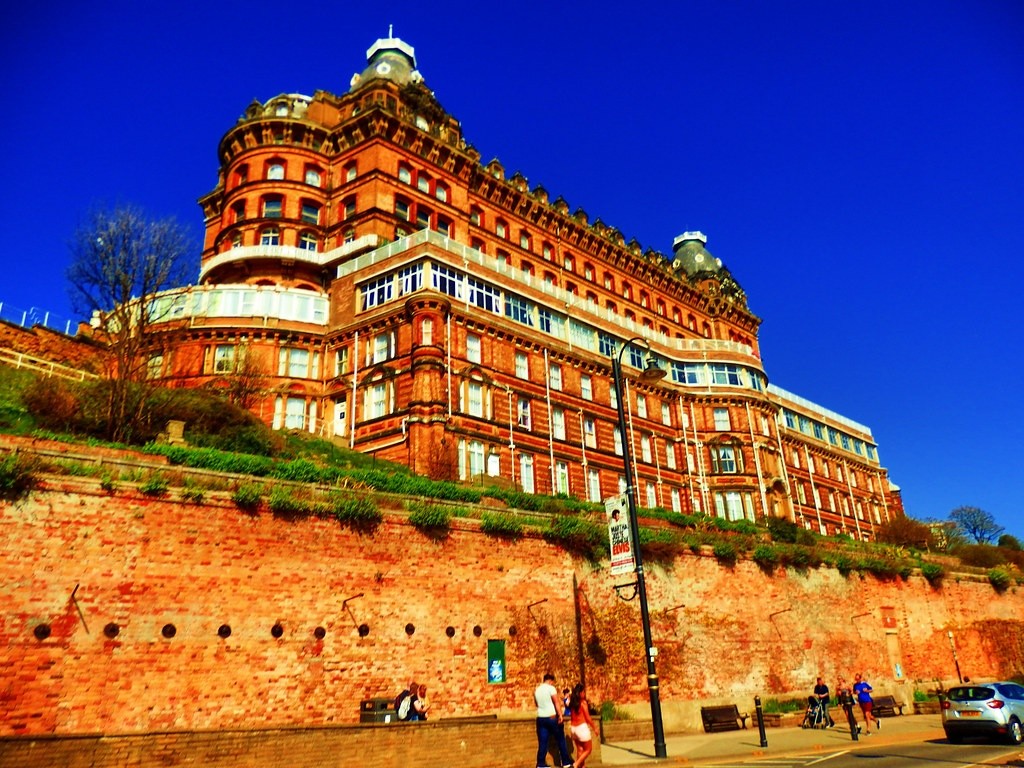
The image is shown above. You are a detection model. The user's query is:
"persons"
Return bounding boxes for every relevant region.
[963,676,972,685]
[570,683,599,768]
[563,688,572,716]
[852,673,881,736]
[401,682,431,722]
[835,675,862,734]
[534,674,573,768]
[810,677,834,728]
[610,510,620,526]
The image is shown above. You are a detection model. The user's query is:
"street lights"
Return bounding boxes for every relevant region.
[612,337,668,758]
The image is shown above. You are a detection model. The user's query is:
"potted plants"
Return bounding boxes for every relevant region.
[912,690,926,714]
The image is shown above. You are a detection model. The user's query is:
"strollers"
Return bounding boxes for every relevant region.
[802,696,825,729]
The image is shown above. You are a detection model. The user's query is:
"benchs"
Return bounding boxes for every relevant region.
[871,695,907,718]
[701,705,750,733]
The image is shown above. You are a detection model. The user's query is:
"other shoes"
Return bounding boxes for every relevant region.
[563,764,573,768]
[826,722,835,728]
[877,718,881,730]
[866,731,872,737]
[536,764,551,768]
[856,726,861,734]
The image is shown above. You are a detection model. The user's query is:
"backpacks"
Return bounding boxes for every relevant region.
[398,693,414,719]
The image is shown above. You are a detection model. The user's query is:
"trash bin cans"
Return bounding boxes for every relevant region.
[360,698,397,722]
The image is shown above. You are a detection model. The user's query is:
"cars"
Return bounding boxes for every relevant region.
[942,682,1024,745]
